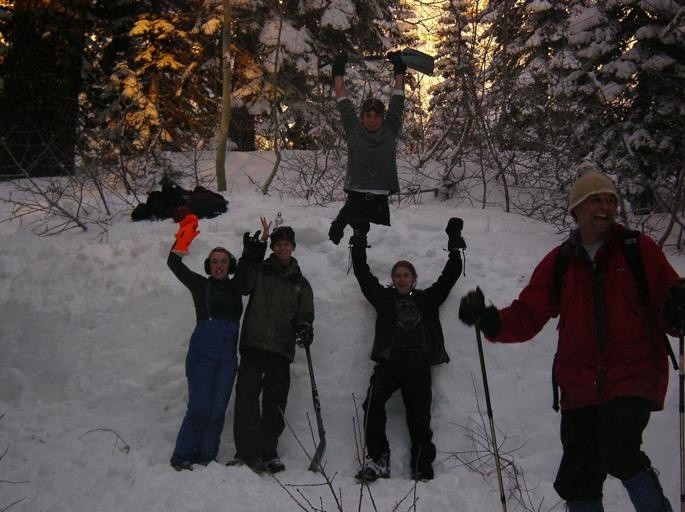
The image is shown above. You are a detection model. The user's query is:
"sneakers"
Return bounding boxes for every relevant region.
[410,453,435,480]
[354,455,391,484]
[225,451,286,474]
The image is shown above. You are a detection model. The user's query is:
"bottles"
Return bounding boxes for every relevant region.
[274,211,283,226]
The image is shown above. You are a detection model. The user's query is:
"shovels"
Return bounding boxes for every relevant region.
[317,46,435,76]
[300,332,328,473]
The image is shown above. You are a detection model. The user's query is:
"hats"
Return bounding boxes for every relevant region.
[567,170,618,214]
[268,225,298,249]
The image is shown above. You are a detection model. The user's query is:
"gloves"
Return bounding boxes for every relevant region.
[293,321,314,349]
[458,285,500,338]
[444,215,466,251]
[331,51,348,76]
[386,51,407,78]
[172,215,199,256]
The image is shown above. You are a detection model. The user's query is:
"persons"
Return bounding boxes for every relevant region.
[328,51,408,245]
[166,215,273,472]
[459,172,685,511]
[346,218,467,482]
[225,225,315,472]
